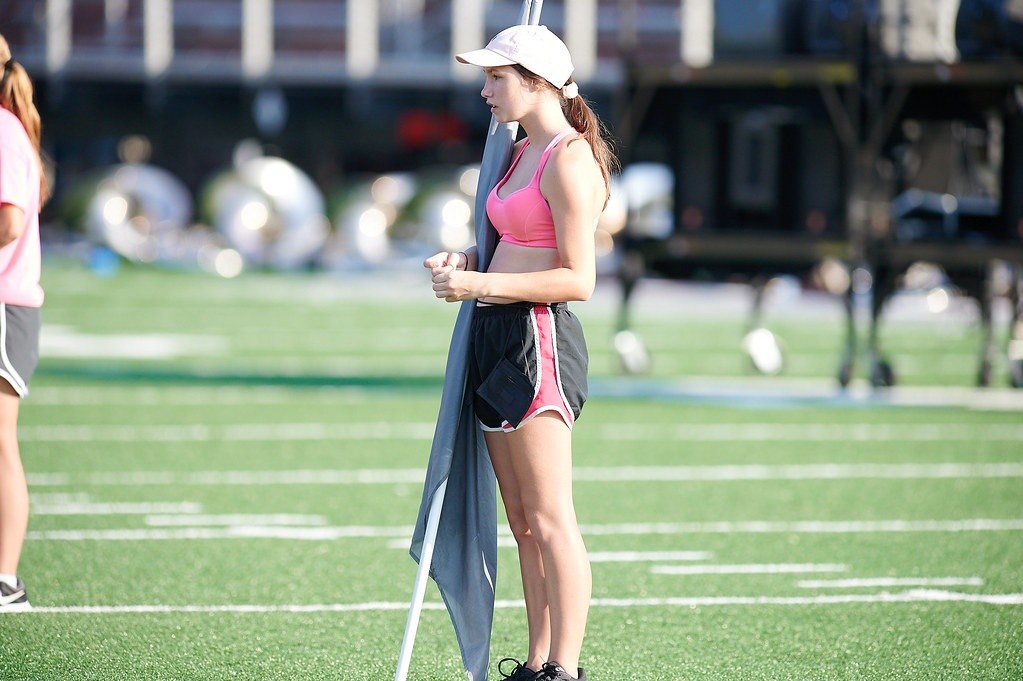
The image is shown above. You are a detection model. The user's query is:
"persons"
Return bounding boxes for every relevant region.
[424,24,613,680]
[0,33,44,610]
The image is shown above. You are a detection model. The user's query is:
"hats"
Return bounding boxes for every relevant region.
[455,24,575,89]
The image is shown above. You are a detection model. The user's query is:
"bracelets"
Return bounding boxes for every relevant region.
[458,252,468,271]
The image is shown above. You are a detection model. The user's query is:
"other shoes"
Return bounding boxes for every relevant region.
[495,658,535,681]
[0,577,31,612]
[527,661,587,681]
